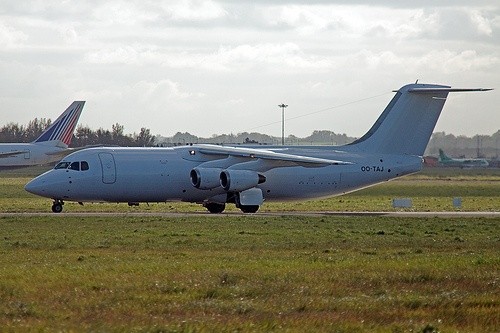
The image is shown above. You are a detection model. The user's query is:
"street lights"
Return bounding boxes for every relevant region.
[278,104,288,146]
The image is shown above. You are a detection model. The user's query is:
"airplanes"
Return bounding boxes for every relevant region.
[0,101,85,167]
[437,147,489,169]
[24,80,495,214]
